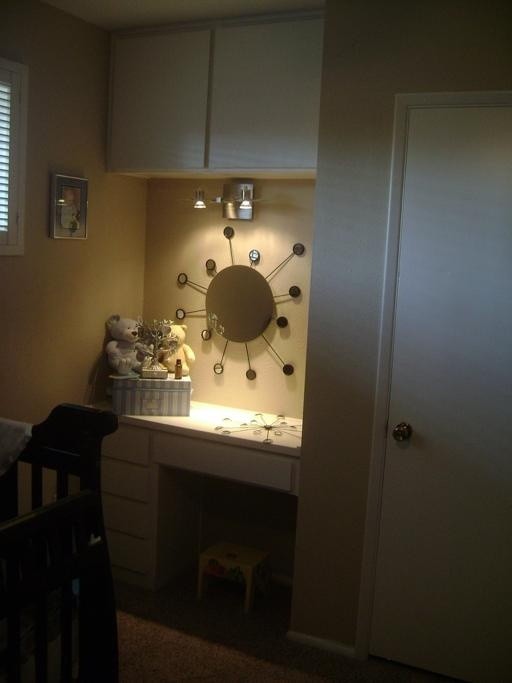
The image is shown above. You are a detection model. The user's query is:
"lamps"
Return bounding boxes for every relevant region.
[190,187,207,209]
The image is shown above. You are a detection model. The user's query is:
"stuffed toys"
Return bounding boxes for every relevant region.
[107,313,145,375]
[161,323,196,376]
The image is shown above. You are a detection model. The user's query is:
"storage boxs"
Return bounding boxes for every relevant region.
[113,373,191,417]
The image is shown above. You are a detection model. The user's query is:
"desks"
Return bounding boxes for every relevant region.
[85,398,302,595]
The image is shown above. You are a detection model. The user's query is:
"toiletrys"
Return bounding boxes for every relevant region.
[174,359,183,380]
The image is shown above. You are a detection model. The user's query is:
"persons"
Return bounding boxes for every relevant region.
[60,189,79,229]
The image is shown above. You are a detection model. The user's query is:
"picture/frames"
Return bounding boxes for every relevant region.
[50,174,88,241]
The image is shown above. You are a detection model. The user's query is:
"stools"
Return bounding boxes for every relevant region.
[197,539,273,614]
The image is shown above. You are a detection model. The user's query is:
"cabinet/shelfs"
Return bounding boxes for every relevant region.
[107,8,324,176]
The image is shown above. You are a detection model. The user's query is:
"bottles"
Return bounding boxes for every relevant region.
[175,358,184,379]
